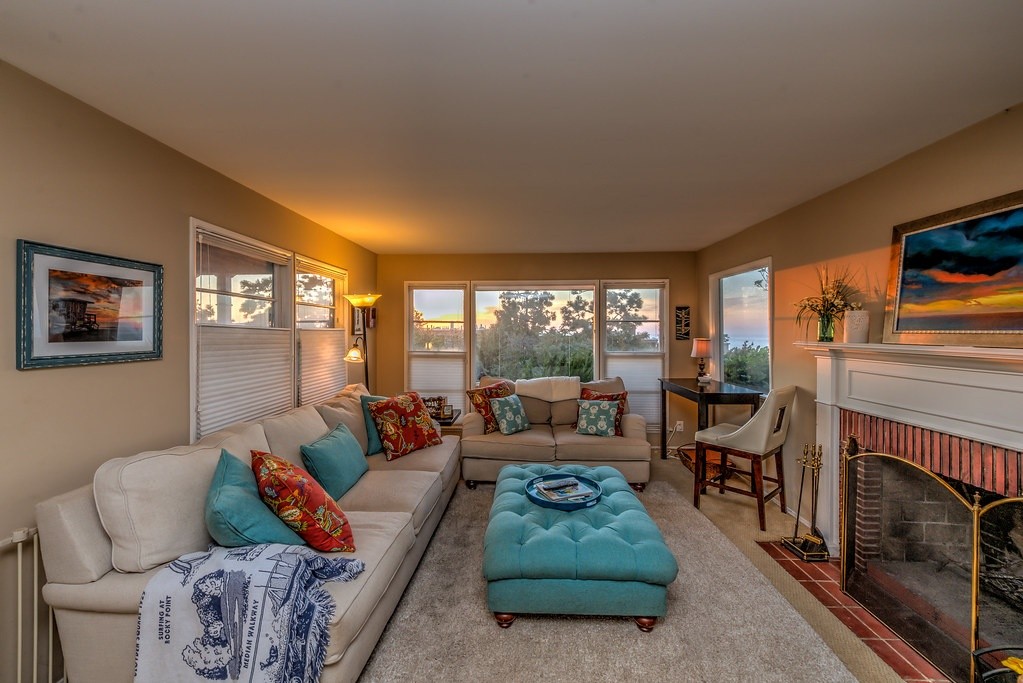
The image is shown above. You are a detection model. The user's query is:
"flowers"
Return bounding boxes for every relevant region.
[846,301,865,311]
[791,262,869,340]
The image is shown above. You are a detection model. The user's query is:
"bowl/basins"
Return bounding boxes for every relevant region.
[698,377,712,382]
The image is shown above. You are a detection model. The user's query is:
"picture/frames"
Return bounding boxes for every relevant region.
[351,304,364,335]
[844,311,870,343]
[881,189,1023,349]
[15,239,164,372]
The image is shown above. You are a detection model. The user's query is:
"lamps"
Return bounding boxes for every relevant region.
[691,338,713,381]
[342,294,382,391]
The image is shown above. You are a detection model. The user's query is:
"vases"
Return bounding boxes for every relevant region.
[817,314,835,342]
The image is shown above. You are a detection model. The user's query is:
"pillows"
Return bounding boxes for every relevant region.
[250,450,356,552]
[205,448,306,550]
[360,395,390,456]
[300,422,369,502]
[577,399,620,437]
[576,388,628,437]
[368,392,443,461]
[465,381,513,435]
[489,393,532,435]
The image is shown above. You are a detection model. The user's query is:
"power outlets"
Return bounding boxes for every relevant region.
[677,421,683,431]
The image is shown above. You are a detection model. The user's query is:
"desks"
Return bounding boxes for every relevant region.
[658,377,763,494]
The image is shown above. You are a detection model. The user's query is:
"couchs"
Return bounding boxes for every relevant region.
[36,382,462,683]
[461,376,652,492]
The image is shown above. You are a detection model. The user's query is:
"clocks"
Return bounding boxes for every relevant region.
[441,405,453,420]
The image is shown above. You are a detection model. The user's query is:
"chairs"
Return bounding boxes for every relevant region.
[694,384,796,531]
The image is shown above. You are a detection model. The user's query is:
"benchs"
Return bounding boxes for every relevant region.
[482,463,679,633]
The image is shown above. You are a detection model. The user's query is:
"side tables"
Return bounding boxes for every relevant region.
[432,409,461,426]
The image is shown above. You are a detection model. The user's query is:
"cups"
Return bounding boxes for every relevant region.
[843,310,869,343]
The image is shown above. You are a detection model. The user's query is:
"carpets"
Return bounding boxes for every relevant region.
[357,472,860,683]
